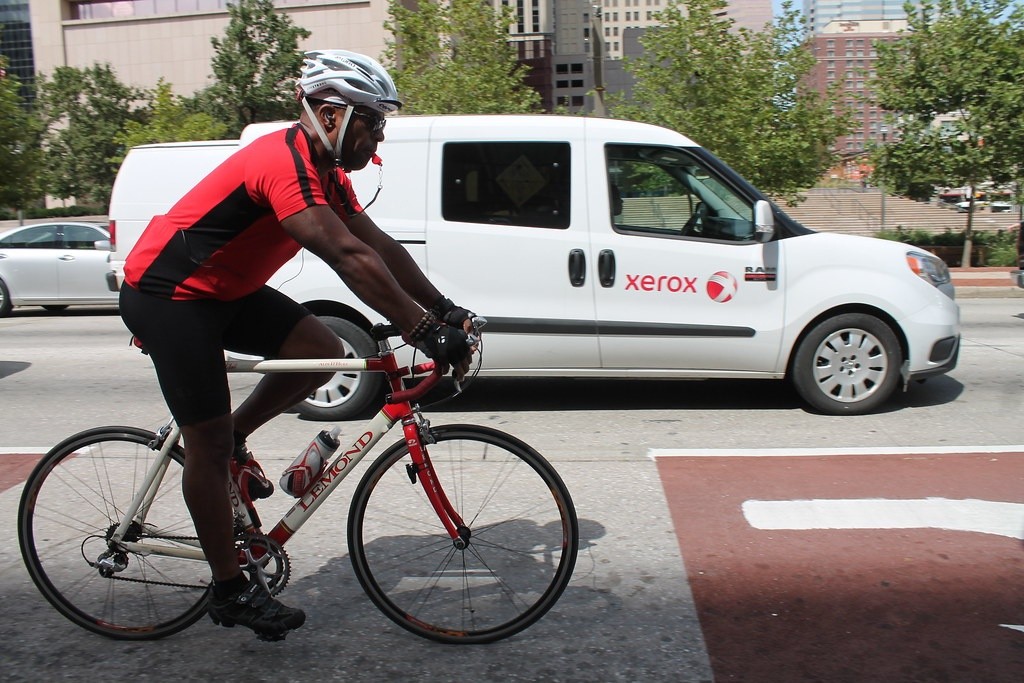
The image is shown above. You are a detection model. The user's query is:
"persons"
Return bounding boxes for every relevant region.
[611,184,624,217]
[126,50,475,639]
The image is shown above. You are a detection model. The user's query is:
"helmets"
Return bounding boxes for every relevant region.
[295,50,402,114]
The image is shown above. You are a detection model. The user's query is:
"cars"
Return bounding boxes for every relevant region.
[0,219,121,318]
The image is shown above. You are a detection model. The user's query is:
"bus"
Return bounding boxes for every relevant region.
[939,187,1013,213]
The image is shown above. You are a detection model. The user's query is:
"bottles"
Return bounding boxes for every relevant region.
[280,427,341,497]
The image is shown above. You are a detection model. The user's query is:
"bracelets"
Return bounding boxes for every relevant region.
[408,312,436,344]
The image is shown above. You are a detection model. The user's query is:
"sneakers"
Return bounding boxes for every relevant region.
[229,442,274,498]
[207,581,306,636]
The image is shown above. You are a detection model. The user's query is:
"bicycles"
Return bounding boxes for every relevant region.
[15,314,582,645]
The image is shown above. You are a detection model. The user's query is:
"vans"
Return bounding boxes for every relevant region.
[230,111,965,424]
[102,139,244,293]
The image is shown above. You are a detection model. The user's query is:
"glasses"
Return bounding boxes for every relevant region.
[333,105,386,131]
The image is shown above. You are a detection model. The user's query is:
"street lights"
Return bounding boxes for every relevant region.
[879,123,892,229]
